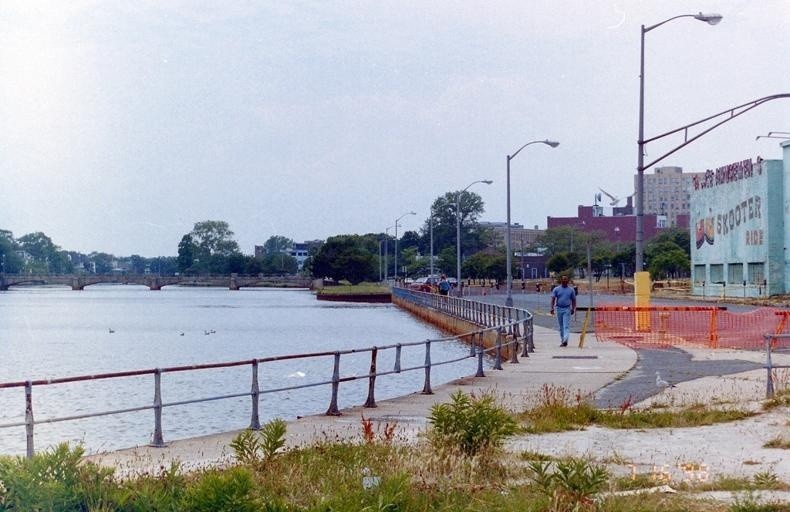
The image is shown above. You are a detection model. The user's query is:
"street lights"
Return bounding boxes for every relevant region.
[634,13,724,334]
[767,131,790,137]
[569,220,586,252]
[506,141,561,304]
[379,211,417,286]
[429,201,456,291]
[455,179,492,300]
[593,192,602,216]
[755,135,790,141]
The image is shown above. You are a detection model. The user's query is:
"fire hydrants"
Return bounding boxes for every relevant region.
[658,311,671,334]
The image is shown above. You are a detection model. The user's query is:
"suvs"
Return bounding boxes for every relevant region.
[412,276,437,291]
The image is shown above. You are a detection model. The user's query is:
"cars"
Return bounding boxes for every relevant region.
[404,277,414,285]
[104,268,128,277]
[446,277,458,287]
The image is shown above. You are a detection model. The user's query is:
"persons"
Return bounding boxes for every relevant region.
[550,275,576,348]
[437,274,450,303]
